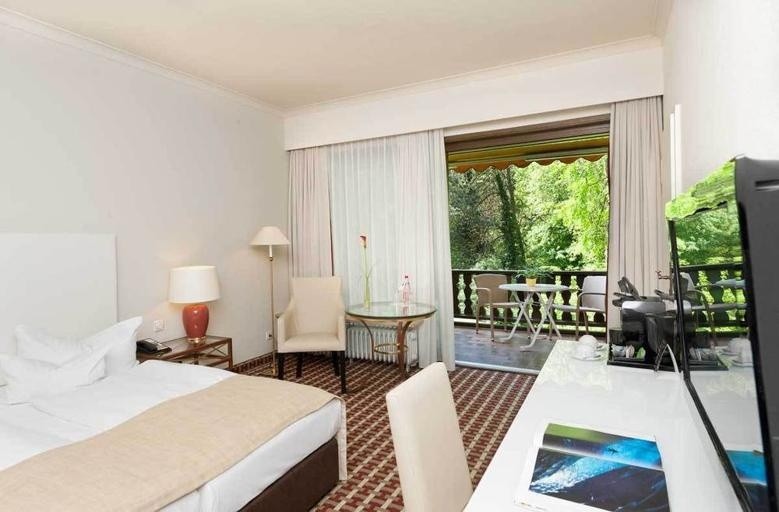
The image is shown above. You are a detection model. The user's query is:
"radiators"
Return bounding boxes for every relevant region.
[312,325,418,373]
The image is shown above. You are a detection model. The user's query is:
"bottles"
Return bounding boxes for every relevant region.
[403,305,409,315]
[402,274,411,304]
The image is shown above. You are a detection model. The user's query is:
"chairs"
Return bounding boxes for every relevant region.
[385,362,473,512]
[679,271,749,346]
[277,278,346,395]
[473,274,531,342]
[548,275,606,341]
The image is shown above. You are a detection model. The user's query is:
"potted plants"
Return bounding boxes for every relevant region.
[516,262,554,286]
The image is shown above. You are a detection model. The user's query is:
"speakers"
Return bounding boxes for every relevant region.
[621,312,647,346]
[647,310,678,353]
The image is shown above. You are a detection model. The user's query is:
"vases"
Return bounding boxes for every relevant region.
[363,276,371,310]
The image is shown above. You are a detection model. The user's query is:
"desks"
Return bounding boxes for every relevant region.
[137,334,233,371]
[463,338,746,512]
[498,283,568,352]
[345,301,438,379]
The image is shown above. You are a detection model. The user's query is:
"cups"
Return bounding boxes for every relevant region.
[578,335,598,356]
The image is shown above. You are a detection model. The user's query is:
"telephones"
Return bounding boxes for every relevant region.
[136,338,171,354]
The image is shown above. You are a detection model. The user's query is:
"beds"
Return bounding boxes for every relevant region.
[0,231,347,511]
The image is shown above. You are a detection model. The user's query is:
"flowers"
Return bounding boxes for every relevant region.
[359,235,370,280]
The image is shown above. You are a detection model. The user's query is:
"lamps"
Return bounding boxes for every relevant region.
[250,226,291,376]
[168,266,222,342]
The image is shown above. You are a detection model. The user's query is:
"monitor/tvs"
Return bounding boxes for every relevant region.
[666,157,779,512]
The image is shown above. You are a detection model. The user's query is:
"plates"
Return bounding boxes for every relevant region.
[720,338,753,368]
[573,344,605,360]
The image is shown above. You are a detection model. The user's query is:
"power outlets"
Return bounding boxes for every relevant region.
[152,320,164,333]
[265,330,273,341]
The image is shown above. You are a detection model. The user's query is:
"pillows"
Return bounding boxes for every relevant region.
[13,324,109,390]
[0,354,65,405]
[95,315,142,371]
